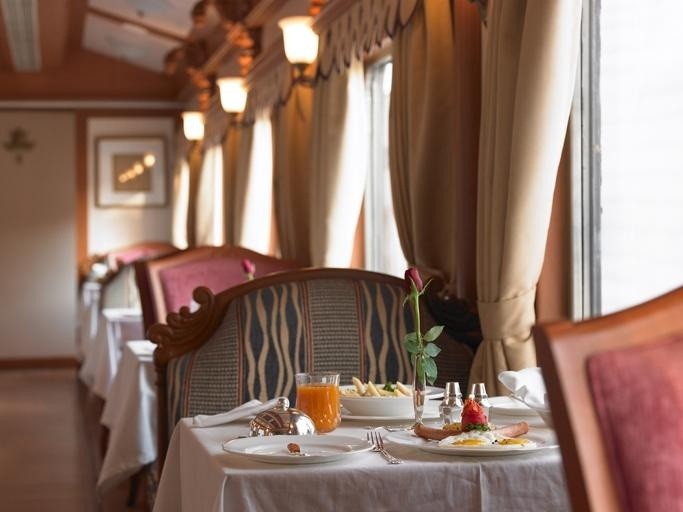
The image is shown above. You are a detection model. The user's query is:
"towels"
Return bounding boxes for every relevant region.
[193,397,278,428]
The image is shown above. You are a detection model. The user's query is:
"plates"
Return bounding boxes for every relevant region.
[376,425,413,446]
[222,434,374,463]
[485,393,538,416]
[339,401,444,420]
[418,433,561,457]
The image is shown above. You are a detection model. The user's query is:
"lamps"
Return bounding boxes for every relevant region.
[181,111,205,141]
[214,76,251,129]
[277,15,319,79]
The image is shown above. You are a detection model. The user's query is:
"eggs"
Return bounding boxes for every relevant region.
[492,438,536,449]
[439,431,492,450]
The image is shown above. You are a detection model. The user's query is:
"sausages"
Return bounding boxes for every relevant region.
[415,422,527,441]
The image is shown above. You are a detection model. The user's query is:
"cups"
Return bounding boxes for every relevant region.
[294,372,341,432]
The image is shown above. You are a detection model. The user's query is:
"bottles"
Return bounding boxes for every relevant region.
[470,383,491,425]
[440,382,462,424]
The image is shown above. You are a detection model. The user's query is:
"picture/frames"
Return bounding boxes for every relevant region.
[95,136,168,209]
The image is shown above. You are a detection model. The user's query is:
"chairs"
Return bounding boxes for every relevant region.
[116,240,182,271]
[146,268,418,484]
[530,285,683,512]
[101,261,142,309]
[127,242,313,506]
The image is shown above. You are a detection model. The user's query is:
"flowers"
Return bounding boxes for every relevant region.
[239,257,257,281]
[401,268,444,387]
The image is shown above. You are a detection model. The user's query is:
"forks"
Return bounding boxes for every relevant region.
[366,432,406,465]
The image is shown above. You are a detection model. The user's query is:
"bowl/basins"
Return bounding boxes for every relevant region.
[340,384,446,415]
